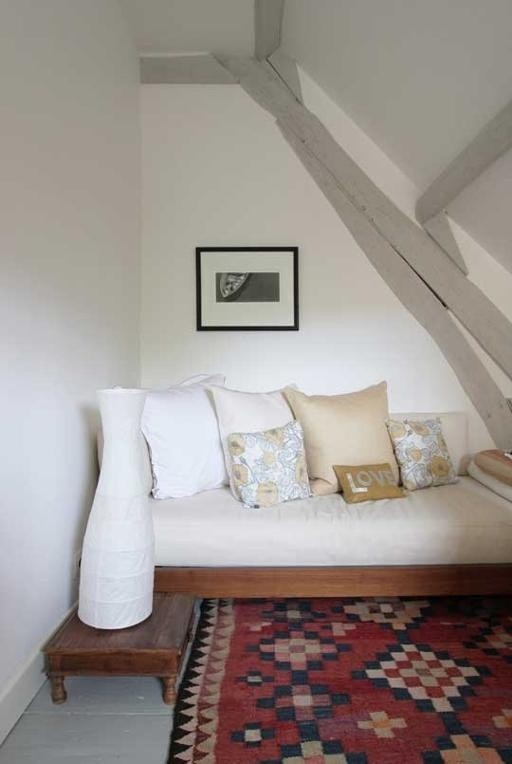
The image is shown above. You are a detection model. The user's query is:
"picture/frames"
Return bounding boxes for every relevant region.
[195,246,299,331]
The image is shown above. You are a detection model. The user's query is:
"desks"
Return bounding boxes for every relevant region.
[40,597,194,702]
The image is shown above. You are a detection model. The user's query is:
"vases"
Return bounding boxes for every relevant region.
[75,387,155,628]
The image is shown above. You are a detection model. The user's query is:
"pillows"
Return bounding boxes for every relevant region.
[228,420,313,509]
[386,417,459,490]
[331,464,409,503]
[208,383,297,482]
[282,381,400,497]
[114,375,227,499]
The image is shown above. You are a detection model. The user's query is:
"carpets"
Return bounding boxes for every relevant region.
[167,597,511,764]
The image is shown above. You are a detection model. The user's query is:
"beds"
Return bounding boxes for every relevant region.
[102,447,512,599]
